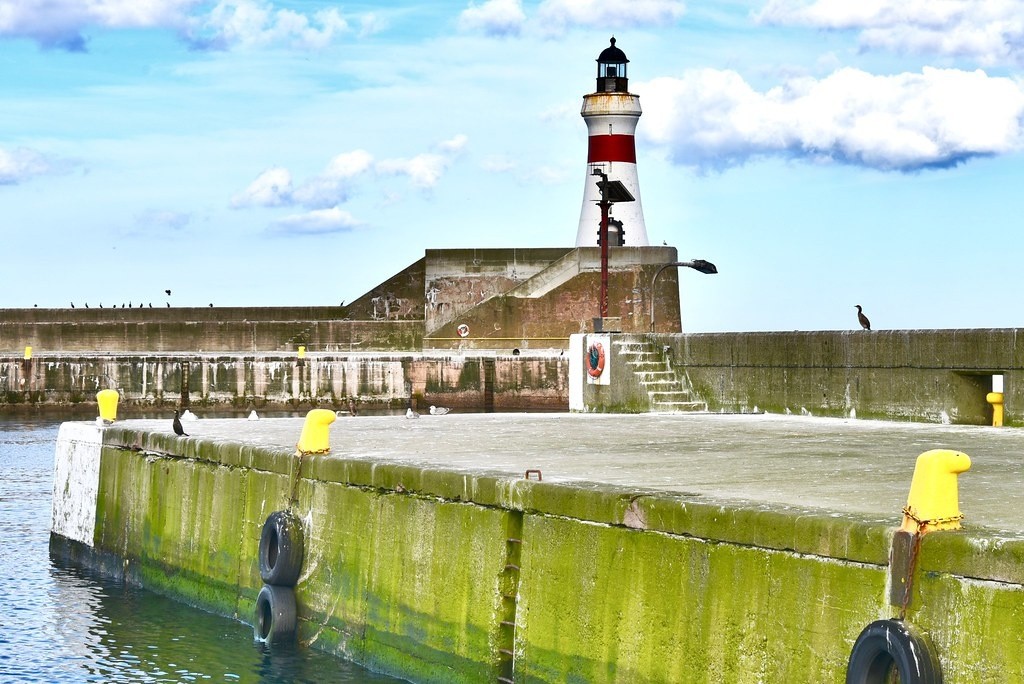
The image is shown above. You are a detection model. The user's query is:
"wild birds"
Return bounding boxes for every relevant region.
[854,305,870,331]
[209,303,213,307]
[172,410,190,437]
[33,289,172,308]
[430,405,450,415]
[405,408,420,419]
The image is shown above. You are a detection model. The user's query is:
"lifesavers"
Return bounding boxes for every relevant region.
[258,508,304,584]
[253,584,298,645]
[845,616,942,684]
[585,341,606,378]
[457,323,470,337]
